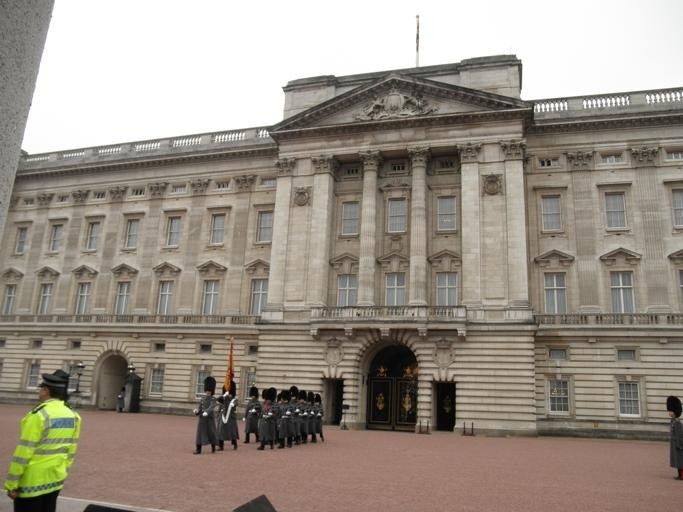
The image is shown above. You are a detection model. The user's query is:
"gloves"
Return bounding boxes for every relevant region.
[193,409,198,414]
[242,409,322,421]
[203,412,208,416]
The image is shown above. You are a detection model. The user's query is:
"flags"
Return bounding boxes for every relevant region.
[224,342,234,390]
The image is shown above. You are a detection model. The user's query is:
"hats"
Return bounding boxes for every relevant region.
[247,386,321,405]
[667,396,681,417]
[38,374,68,388]
[204,376,216,395]
[223,380,236,398]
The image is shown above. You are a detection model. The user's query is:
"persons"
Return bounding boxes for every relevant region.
[3,370,82,512]
[193,376,325,454]
[117,387,126,413]
[666,396,683,480]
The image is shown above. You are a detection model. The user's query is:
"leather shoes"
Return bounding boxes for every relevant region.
[192,446,237,454]
[244,436,326,450]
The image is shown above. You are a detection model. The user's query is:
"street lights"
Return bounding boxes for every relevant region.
[73,361,85,394]
